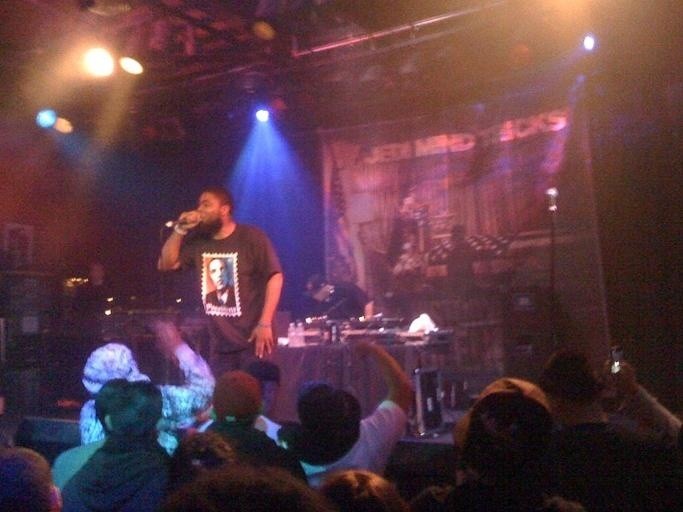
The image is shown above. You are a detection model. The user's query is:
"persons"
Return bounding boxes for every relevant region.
[0,184,683,512]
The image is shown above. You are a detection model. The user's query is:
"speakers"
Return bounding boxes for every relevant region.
[16,416,81,466]
[385,440,458,480]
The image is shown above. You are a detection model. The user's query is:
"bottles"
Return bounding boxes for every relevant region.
[287,321,305,347]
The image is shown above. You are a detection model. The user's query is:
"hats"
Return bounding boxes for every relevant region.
[94,377,162,436]
[81,343,151,394]
[246,360,280,382]
[298,384,361,442]
[212,370,261,416]
[300,274,326,296]
[453,377,555,444]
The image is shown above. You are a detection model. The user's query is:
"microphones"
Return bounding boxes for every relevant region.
[166,218,186,228]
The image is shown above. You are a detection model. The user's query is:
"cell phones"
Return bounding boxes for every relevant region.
[611,343,625,375]
